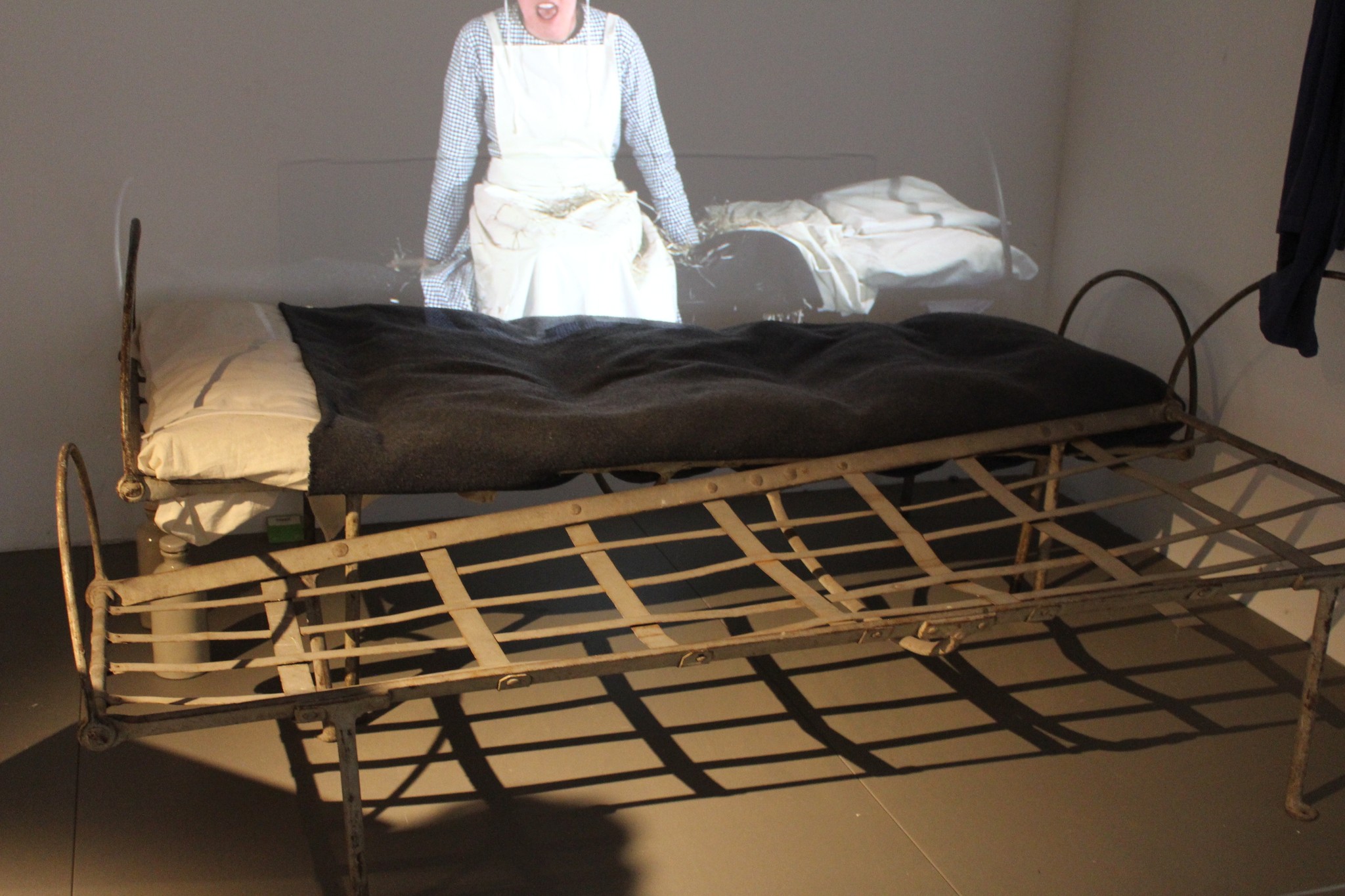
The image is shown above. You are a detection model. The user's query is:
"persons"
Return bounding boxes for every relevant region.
[420,0,699,325]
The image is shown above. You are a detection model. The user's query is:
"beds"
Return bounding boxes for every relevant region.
[55,219,1200,739]
[112,134,1039,320]
